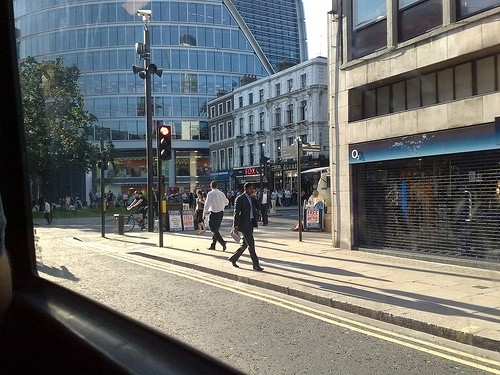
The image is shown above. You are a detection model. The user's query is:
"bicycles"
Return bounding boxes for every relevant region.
[121,208,157,232]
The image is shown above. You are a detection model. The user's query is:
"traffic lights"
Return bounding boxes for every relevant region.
[158,124,172,160]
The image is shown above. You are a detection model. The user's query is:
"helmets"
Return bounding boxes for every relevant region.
[134,191,139,193]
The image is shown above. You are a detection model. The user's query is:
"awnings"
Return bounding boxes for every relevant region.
[300,167,329,173]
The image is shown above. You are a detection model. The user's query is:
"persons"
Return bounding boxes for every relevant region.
[308,190,321,207]
[59,194,82,211]
[193,190,206,234]
[168,187,227,214]
[127,191,148,231]
[227,185,297,226]
[228,182,262,272]
[32,192,49,211]
[0,196,12,310]
[201,181,229,252]
[89,189,133,209]
[43,199,53,224]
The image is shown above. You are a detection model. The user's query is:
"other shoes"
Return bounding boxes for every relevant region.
[254,265,262,271]
[222,242,226,251]
[209,246,215,250]
[228,257,239,267]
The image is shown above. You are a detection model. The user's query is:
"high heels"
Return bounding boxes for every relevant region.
[198,230,204,234]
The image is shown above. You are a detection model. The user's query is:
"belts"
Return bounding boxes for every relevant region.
[250,219,255,221]
